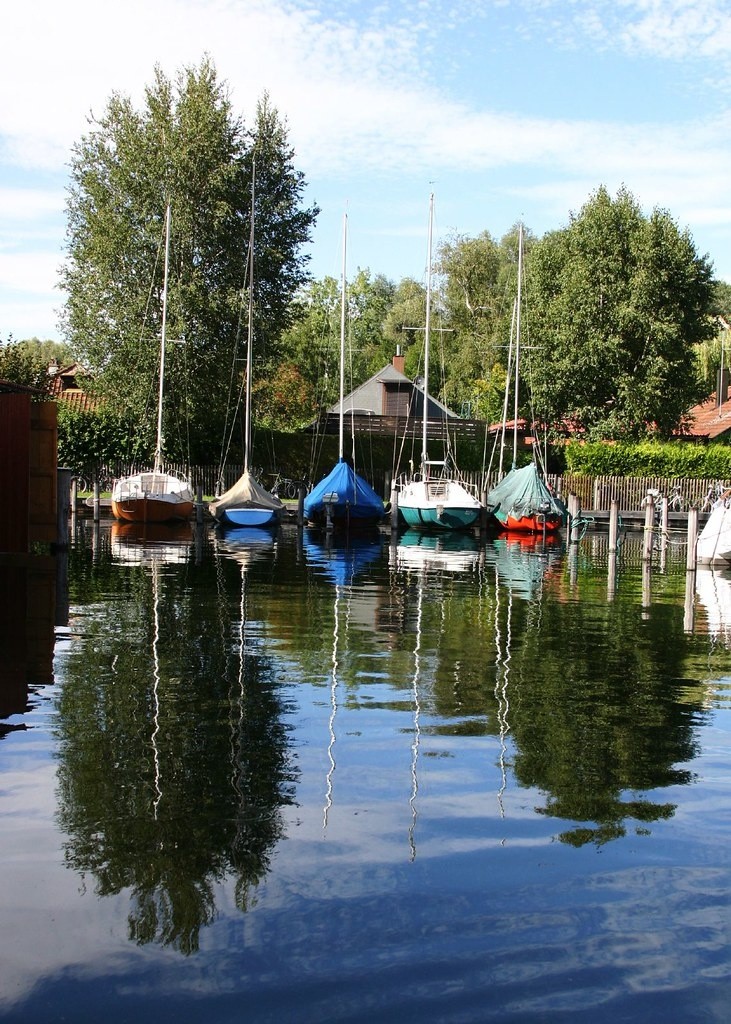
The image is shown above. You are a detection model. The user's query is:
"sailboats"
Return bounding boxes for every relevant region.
[297,211,388,525]
[206,155,291,524]
[391,190,484,533]
[110,203,197,524]
[499,223,566,534]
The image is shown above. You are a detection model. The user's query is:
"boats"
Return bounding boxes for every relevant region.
[696,490,731,566]
